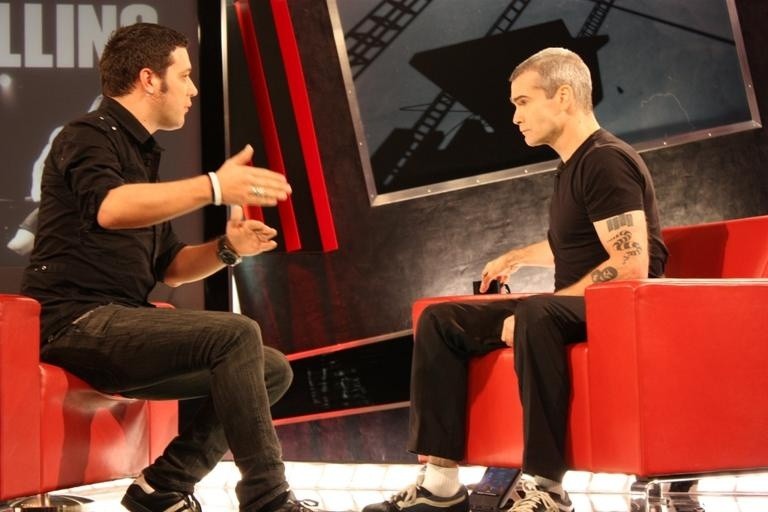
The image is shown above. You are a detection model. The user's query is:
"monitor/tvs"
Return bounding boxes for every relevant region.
[326,0,763,208]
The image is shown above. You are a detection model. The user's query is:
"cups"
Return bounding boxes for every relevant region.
[473,279,500,295]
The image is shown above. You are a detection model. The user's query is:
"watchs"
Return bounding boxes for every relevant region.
[217,234,243,268]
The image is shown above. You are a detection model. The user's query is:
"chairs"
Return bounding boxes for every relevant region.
[410,215,768,512]
[1,296,180,512]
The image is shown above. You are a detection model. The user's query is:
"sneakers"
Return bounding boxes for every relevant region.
[362,475,469,512]
[261,491,319,512]
[121,470,202,512]
[501,475,575,511]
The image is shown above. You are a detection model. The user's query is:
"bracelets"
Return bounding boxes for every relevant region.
[207,169,222,207]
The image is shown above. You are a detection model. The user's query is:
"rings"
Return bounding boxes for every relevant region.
[252,185,266,196]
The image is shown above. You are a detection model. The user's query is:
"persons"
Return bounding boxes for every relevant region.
[22,22,325,512]
[362,46,669,512]
